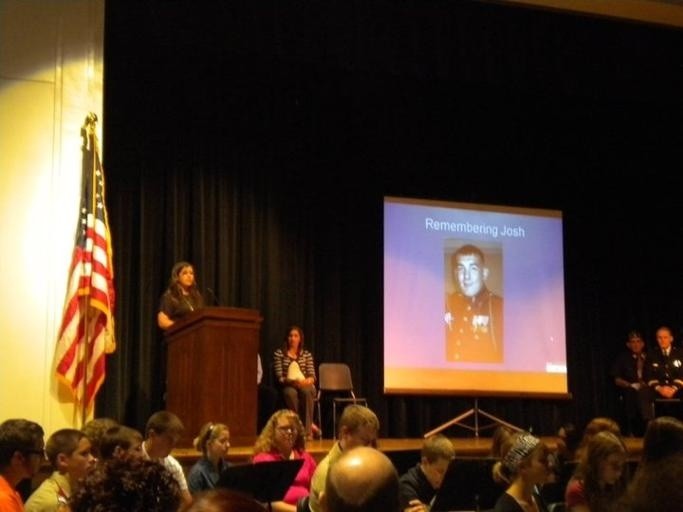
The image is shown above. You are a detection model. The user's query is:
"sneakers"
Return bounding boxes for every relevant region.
[314,429,322,438]
[306,431,313,441]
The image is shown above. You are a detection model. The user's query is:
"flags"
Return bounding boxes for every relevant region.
[48,124,120,418]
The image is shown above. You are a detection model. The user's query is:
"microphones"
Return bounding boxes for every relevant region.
[207,288,220,304]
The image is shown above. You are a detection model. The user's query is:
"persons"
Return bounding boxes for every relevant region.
[309,404,380,510]
[156,259,208,333]
[272,323,327,441]
[442,240,503,364]
[185,488,265,510]
[251,409,317,511]
[492,416,682,511]
[609,326,655,402]
[399,435,456,510]
[186,422,232,500]
[641,326,682,400]
[319,446,400,510]
[1,411,193,510]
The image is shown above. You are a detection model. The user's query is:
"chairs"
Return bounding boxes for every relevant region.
[313,362,369,440]
